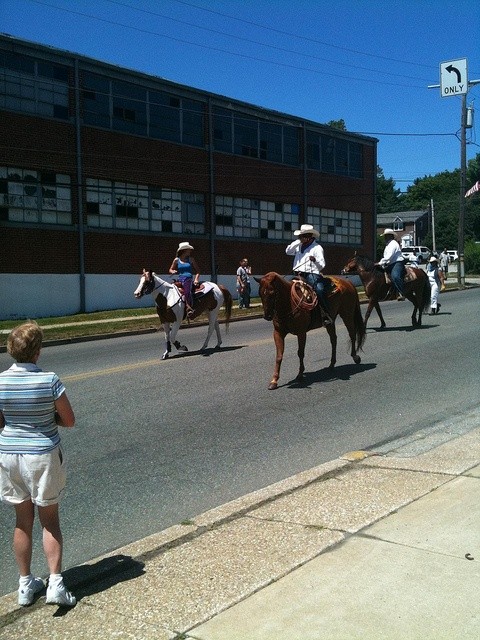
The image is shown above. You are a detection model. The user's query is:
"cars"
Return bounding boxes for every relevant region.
[447,250,458,261]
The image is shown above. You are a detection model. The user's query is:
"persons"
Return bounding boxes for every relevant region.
[286,224,334,327]
[409,248,419,269]
[425,257,445,316]
[236,260,248,309]
[439,248,450,280]
[244,258,251,306]
[1,320,75,607]
[169,242,200,317]
[374,228,407,301]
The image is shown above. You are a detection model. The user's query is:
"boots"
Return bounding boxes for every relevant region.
[437,304,441,312]
[431,308,435,315]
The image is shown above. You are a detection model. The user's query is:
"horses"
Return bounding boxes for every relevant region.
[133,268,233,360]
[342,251,431,328]
[253,272,367,390]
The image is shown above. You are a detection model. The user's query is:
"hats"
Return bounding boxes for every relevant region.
[177,241,195,253]
[427,256,439,262]
[293,224,321,238]
[379,228,399,240]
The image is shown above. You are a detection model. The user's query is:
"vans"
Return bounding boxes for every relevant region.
[401,246,432,264]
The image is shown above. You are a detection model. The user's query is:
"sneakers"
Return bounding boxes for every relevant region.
[45,584,78,607]
[17,576,44,605]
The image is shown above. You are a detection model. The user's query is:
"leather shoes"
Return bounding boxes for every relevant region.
[187,306,194,316]
[323,314,334,320]
[397,295,406,301]
